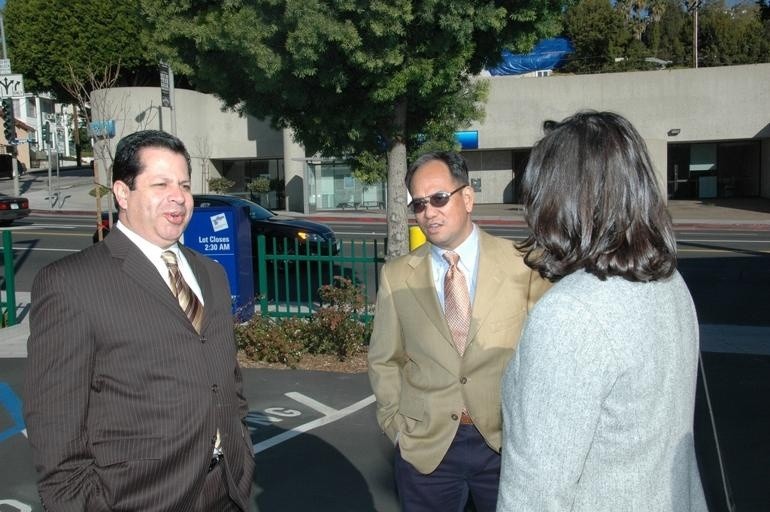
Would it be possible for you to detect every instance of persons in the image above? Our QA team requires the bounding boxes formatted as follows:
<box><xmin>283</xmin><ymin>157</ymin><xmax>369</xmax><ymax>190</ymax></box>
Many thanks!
<box><xmin>367</xmin><ymin>149</ymin><xmax>557</xmax><ymax>512</ymax></box>
<box><xmin>24</xmin><ymin>129</ymin><xmax>266</xmax><ymax>512</ymax></box>
<box><xmin>492</xmin><ymin>110</ymin><xmax>710</xmax><ymax>512</ymax></box>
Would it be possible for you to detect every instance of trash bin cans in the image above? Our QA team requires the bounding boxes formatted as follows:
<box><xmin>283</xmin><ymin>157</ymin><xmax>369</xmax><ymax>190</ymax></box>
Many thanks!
<box><xmin>182</xmin><ymin>204</ymin><xmax>255</xmax><ymax>325</ymax></box>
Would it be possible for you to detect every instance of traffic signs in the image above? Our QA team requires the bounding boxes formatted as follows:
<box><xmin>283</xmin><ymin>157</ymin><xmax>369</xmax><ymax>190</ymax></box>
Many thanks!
<box><xmin>0</xmin><ymin>58</ymin><xmax>12</xmax><ymax>75</ymax></box>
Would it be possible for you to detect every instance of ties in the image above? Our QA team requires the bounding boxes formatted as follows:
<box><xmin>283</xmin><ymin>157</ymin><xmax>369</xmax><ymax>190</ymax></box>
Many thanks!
<box><xmin>159</xmin><ymin>250</ymin><xmax>203</xmax><ymax>334</ymax></box>
<box><xmin>441</xmin><ymin>252</ymin><xmax>472</xmax><ymax>356</ymax></box>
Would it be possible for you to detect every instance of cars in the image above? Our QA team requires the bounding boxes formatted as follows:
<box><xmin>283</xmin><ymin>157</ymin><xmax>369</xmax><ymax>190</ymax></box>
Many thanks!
<box><xmin>0</xmin><ymin>190</ymin><xmax>33</xmax><ymax>228</ymax></box>
<box><xmin>0</xmin><ymin>153</ymin><xmax>25</xmax><ymax>180</ymax></box>
<box><xmin>90</xmin><ymin>191</ymin><xmax>342</xmax><ymax>281</ymax></box>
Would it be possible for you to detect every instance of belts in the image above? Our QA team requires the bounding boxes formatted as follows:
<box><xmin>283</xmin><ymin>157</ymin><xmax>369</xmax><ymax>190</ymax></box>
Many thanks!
<box><xmin>208</xmin><ymin>454</ymin><xmax>225</xmax><ymax>471</ymax></box>
<box><xmin>460</xmin><ymin>412</ymin><xmax>473</xmax><ymax>426</ymax></box>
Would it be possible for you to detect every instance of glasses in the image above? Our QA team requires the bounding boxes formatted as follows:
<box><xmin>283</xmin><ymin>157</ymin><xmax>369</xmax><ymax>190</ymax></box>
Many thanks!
<box><xmin>407</xmin><ymin>183</ymin><xmax>468</xmax><ymax>214</ymax></box>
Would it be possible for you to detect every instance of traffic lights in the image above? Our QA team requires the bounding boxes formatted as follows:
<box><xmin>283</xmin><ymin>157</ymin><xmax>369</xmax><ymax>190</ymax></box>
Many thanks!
<box><xmin>41</xmin><ymin>121</ymin><xmax>50</xmax><ymax>143</ymax></box>
<box><xmin>2</xmin><ymin>98</ymin><xmax>17</xmax><ymax>143</ymax></box>
